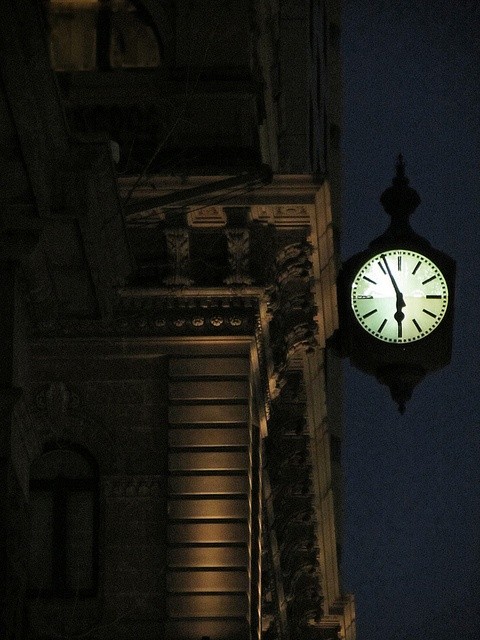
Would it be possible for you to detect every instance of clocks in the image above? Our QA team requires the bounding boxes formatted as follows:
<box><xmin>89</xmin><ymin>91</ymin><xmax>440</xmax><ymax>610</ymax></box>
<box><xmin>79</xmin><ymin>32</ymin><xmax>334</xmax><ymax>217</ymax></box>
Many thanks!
<box><xmin>328</xmin><ymin>154</ymin><xmax>457</xmax><ymax>413</ymax></box>
